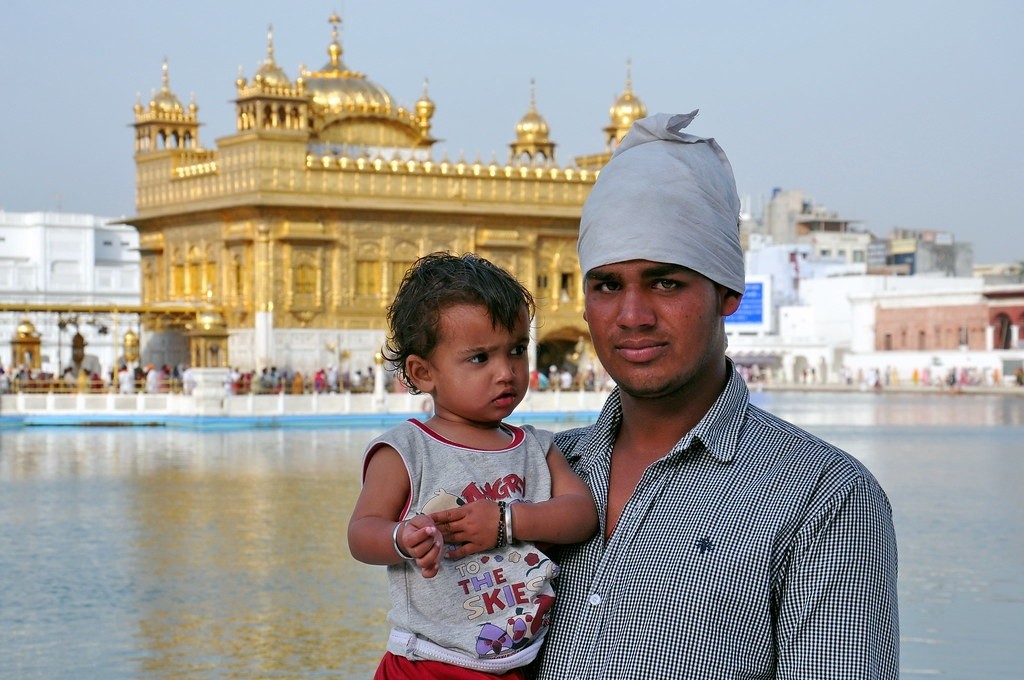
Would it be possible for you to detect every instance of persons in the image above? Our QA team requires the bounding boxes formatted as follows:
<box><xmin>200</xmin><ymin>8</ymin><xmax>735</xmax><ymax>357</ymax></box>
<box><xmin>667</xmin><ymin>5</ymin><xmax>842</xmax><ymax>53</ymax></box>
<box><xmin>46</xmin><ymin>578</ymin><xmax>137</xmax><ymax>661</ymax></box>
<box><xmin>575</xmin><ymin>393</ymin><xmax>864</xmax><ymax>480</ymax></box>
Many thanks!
<box><xmin>1</xmin><ymin>349</ymin><xmax>1024</xmax><ymax>393</ymax></box>
<box><xmin>342</xmin><ymin>250</ymin><xmax>601</xmax><ymax>680</ymax></box>
<box><xmin>520</xmin><ymin>107</ymin><xmax>900</xmax><ymax>680</ymax></box>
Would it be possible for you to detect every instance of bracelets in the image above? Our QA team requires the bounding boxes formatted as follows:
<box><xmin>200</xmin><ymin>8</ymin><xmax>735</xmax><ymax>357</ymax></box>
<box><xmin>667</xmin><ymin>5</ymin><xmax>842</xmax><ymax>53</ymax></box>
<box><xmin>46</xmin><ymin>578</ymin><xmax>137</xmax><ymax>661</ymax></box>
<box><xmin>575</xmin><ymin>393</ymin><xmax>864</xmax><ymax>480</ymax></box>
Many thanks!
<box><xmin>391</xmin><ymin>522</ymin><xmax>414</xmax><ymax>562</ymax></box>
<box><xmin>496</xmin><ymin>497</ymin><xmax>507</xmax><ymax>549</ymax></box>
<box><xmin>503</xmin><ymin>497</ymin><xmax>523</xmax><ymax>544</ymax></box>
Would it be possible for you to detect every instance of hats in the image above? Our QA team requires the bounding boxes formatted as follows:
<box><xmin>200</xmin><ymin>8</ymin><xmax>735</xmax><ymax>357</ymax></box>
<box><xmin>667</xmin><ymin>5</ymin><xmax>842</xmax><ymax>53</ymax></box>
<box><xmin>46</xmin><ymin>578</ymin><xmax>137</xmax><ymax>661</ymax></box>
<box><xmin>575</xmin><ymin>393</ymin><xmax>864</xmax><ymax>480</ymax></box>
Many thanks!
<box><xmin>576</xmin><ymin>108</ymin><xmax>745</xmax><ymax>295</ymax></box>
